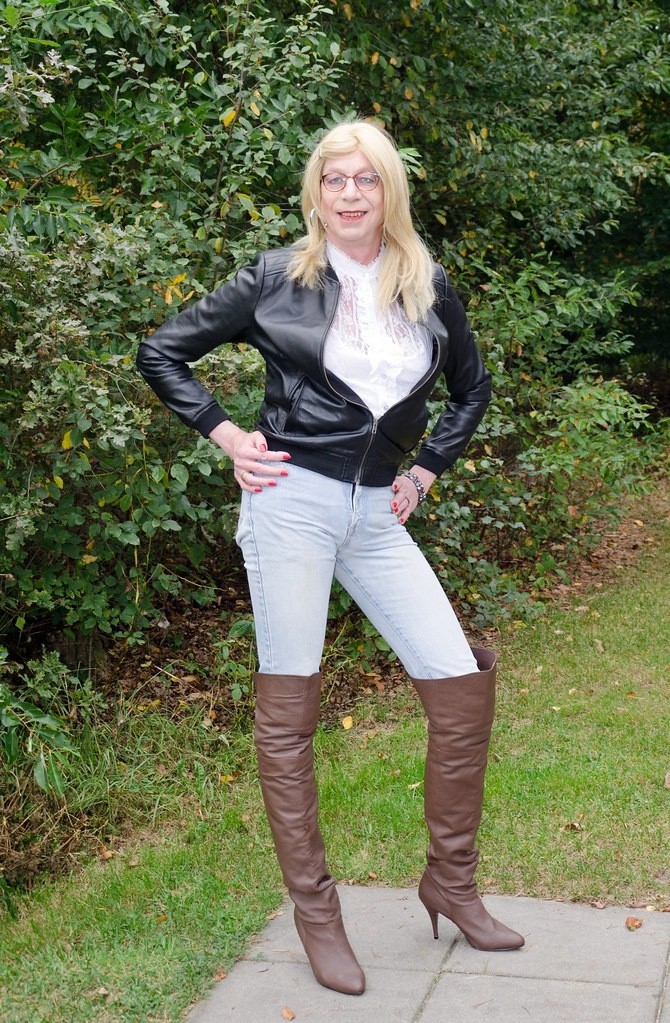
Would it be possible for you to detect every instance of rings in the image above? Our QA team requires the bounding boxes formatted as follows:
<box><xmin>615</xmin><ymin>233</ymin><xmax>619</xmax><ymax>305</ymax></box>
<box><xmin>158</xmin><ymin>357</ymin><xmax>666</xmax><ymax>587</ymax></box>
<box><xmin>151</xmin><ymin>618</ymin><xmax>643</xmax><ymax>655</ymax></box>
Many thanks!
<box><xmin>240</xmin><ymin>472</ymin><xmax>249</xmax><ymax>481</ymax></box>
<box><xmin>406</xmin><ymin>497</ymin><xmax>410</xmax><ymax>503</ymax></box>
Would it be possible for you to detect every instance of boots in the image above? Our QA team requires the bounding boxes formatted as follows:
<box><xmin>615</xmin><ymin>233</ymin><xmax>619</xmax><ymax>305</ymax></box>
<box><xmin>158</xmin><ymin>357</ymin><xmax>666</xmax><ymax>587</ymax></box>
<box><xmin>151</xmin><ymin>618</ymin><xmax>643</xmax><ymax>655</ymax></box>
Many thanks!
<box><xmin>404</xmin><ymin>646</ymin><xmax>526</xmax><ymax>952</ymax></box>
<box><xmin>251</xmin><ymin>668</ymin><xmax>368</xmax><ymax>997</ymax></box>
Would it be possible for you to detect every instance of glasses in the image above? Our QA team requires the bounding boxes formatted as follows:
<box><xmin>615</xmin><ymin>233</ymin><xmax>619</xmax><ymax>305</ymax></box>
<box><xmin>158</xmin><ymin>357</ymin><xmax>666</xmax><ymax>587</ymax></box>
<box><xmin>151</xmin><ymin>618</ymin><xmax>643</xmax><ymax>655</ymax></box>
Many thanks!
<box><xmin>320</xmin><ymin>172</ymin><xmax>380</xmax><ymax>193</ymax></box>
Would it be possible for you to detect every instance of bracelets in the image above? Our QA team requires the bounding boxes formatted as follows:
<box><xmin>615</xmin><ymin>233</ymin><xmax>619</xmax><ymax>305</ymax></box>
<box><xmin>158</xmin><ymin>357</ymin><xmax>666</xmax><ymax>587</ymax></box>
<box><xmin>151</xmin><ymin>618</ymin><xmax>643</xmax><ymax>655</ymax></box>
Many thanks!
<box><xmin>406</xmin><ymin>472</ymin><xmax>427</xmax><ymax>504</ymax></box>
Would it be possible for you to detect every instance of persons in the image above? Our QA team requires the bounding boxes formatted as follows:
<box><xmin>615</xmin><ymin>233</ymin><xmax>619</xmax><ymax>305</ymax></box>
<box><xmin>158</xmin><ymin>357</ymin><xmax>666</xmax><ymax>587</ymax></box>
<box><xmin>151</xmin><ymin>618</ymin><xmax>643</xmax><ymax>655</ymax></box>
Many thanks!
<box><xmin>134</xmin><ymin>123</ymin><xmax>525</xmax><ymax>998</ymax></box>
